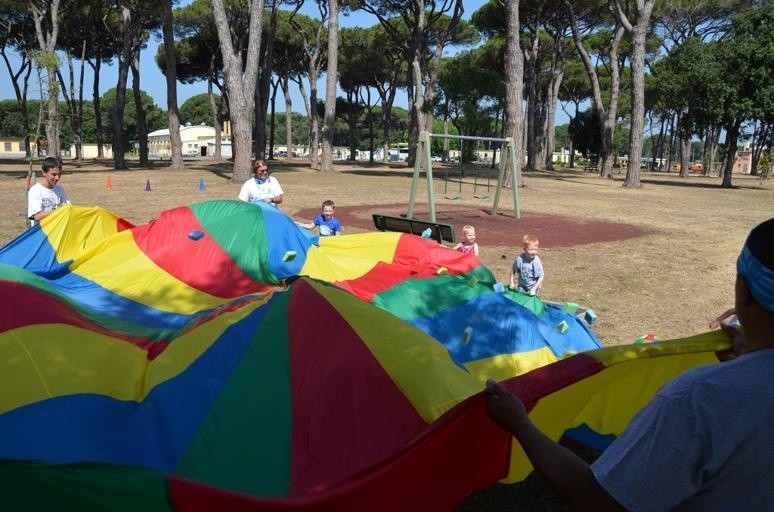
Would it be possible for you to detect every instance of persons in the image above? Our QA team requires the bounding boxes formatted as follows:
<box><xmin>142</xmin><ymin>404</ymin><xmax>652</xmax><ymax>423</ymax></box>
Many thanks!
<box><xmin>507</xmin><ymin>234</ymin><xmax>544</xmax><ymax>299</ymax></box>
<box><xmin>236</xmin><ymin>159</ymin><xmax>282</xmax><ymax>210</ymax></box>
<box><xmin>477</xmin><ymin>216</ymin><xmax>774</xmax><ymax>510</ymax></box>
<box><xmin>293</xmin><ymin>199</ymin><xmax>341</xmax><ymax>236</ymax></box>
<box><xmin>439</xmin><ymin>224</ymin><xmax>479</xmax><ymax>255</ymax></box>
<box><xmin>25</xmin><ymin>157</ymin><xmax>71</xmax><ymax>229</ymax></box>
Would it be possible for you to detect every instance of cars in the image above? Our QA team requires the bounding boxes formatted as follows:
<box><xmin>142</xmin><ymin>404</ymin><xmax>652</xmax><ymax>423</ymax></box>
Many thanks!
<box><xmin>181</xmin><ymin>150</ymin><xmax>198</xmax><ymax>157</ymax></box>
<box><xmin>263</xmin><ymin>149</ymin><xmax>304</xmax><ymax>159</ymax></box>
<box><xmin>430</xmin><ymin>156</ymin><xmax>459</xmax><ymax>163</ymax></box>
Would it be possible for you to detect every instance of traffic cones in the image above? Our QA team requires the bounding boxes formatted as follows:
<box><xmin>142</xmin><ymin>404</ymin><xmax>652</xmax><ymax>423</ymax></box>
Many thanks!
<box><xmin>144</xmin><ymin>179</ymin><xmax>152</xmax><ymax>193</ymax></box>
<box><xmin>104</xmin><ymin>175</ymin><xmax>114</xmax><ymax>192</ymax></box>
<box><xmin>198</xmin><ymin>177</ymin><xmax>206</xmax><ymax>193</ymax></box>
<box><xmin>22</xmin><ymin>175</ymin><xmax>32</xmax><ymax>189</ymax></box>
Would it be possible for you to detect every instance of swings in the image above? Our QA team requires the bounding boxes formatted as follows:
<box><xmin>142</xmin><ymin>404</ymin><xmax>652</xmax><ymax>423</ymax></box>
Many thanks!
<box><xmin>472</xmin><ymin>140</ymin><xmax>491</xmax><ymax>199</ymax></box>
<box><xmin>443</xmin><ymin>138</ymin><xmax>464</xmax><ymax>201</ymax></box>
